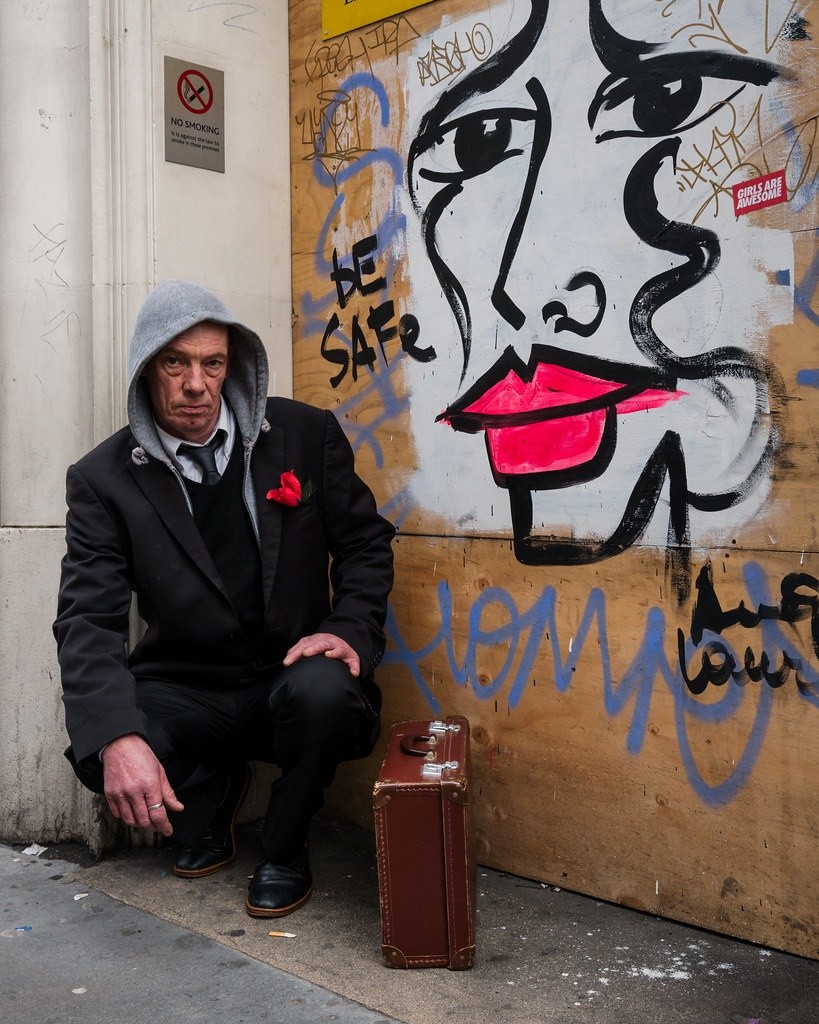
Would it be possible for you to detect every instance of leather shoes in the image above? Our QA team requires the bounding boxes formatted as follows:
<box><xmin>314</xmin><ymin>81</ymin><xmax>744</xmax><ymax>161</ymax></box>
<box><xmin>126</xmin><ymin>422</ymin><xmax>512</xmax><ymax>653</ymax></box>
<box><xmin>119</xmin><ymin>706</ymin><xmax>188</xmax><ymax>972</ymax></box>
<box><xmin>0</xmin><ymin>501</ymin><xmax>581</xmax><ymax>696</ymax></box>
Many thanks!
<box><xmin>246</xmin><ymin>831</ymin><xmax>314</xmax><ymax>917</ymax></box>
<box><xmin>173</xmin><ymin>761</ymin><xmax>252</xmax><ymax>877</ymax></box>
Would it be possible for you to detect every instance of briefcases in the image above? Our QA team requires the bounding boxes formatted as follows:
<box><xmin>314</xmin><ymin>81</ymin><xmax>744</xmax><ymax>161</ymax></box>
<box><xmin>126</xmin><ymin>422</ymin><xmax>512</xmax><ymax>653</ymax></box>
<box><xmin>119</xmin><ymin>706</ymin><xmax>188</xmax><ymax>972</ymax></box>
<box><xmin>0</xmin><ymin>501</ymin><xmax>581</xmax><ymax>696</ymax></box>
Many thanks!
<box><xmin>372</xmin><ymin>714</ymin><xmax>477</xmax><ymax>974</ymax></box>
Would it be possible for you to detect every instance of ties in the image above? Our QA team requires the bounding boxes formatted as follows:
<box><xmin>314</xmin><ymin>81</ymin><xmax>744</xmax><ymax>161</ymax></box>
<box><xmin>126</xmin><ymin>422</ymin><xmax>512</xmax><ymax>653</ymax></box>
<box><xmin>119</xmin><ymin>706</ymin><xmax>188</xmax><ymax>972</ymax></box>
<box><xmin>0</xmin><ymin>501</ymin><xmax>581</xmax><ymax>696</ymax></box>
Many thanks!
<box><xmin>176</xmin><ymin>429</ymin><xmax>229</xmax><ymax>487</ymax></box>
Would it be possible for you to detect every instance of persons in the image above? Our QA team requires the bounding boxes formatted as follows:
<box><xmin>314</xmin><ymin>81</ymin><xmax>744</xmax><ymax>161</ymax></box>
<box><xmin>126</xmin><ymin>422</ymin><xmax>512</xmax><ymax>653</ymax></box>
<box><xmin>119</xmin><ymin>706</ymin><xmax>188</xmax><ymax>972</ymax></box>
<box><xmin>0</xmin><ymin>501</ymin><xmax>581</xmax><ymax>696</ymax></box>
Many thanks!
<box><xmin>50</xmin><ymin>280</ymin><xmax>394</xmax><ymax>918</ymax></box>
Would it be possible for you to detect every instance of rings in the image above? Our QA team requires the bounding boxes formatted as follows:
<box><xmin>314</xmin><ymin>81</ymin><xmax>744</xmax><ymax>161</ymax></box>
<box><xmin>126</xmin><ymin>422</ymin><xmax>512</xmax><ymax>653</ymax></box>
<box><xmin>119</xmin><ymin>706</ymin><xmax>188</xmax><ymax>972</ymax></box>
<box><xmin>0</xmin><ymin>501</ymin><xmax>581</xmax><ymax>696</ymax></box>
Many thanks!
<box><xmin>148</xmin><ymin>802</ymin><xmax>163</xmax><ymax>811</ymax></box>
